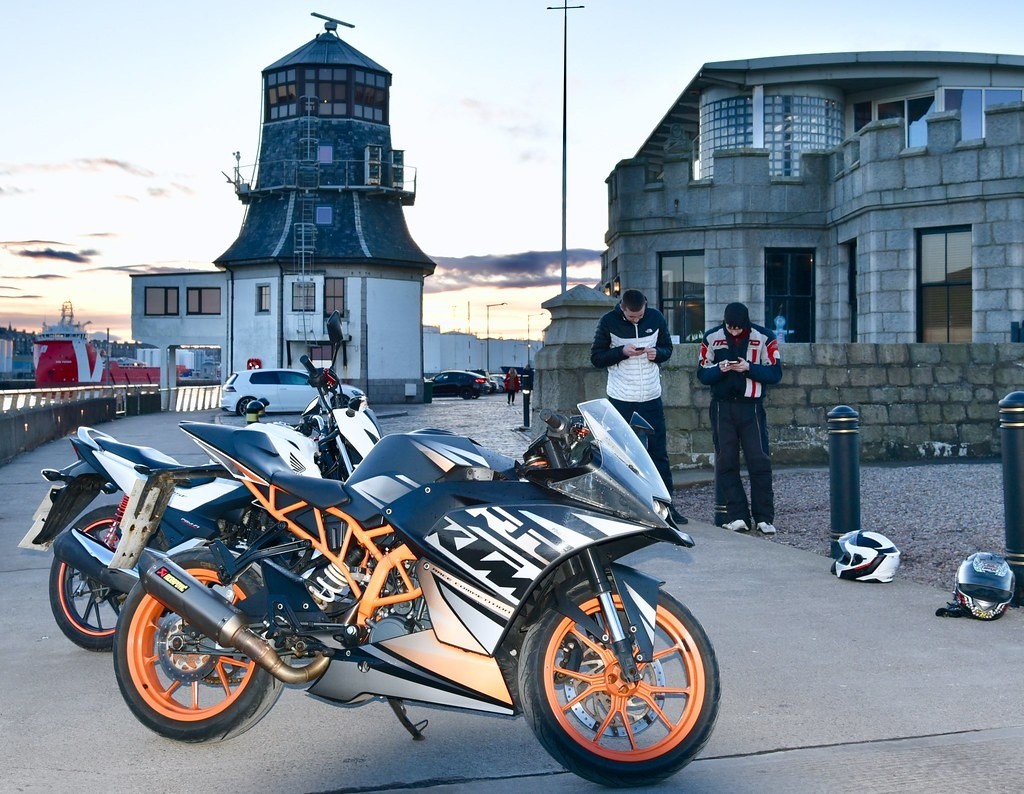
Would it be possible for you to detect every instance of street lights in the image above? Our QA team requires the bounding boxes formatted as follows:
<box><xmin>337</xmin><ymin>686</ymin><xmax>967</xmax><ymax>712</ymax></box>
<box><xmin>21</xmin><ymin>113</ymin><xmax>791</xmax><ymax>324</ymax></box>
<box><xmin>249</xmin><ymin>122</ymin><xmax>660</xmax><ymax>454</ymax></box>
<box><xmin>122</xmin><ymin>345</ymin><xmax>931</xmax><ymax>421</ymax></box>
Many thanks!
<box><xmin>486</xmin><ymin>301</ymin><xmax>508</xmax><ymax>374</ymax></box>
<box><xmin>527</xmin><ymin>312</ymin><xmax>544</xmax><ymax>367</ymax></box>
<box><xmin>546</xmin><ymin>0</ymin><xmax>586</xmax><ymax>294</ymax></box>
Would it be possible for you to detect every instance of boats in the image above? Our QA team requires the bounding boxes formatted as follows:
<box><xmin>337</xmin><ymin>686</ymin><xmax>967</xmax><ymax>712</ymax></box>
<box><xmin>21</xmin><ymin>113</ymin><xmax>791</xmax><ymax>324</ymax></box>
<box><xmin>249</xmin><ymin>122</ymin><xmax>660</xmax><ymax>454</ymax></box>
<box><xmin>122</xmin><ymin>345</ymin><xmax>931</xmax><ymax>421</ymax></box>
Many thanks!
<box><xmin>27</xmin><ymin>301</ymin><xmax>181</xmax><ymax>402</ymax></box>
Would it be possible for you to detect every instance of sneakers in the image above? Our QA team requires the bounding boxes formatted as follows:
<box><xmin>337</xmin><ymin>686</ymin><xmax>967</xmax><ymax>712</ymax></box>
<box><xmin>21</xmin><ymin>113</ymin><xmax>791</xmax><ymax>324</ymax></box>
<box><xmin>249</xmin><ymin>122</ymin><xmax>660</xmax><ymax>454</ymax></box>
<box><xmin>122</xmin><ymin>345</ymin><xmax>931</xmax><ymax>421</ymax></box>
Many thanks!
<box><xmin>756</xmin><ymin>522</ymin><xmax>776</xmax><ymax>534</ymax></box>
<box><xmin>723</xmin><ymin>519</ymin><xmax>749</xmax><ymax>531</ymax></box>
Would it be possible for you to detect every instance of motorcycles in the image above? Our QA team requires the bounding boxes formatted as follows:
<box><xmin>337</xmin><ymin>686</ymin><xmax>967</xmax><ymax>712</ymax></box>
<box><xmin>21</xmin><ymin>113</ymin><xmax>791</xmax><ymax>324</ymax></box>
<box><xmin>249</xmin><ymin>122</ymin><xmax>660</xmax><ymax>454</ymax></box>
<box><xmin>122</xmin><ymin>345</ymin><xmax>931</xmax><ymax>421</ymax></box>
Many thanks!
<box><xmin>110</xmin><ymin>399</ymin><xmax>722</xmax><ymax>788</ymax></box>
<box><xmin>16</xmin><ymin>309</ymin><xmax>385</xmax><ymax>653</ymax></box>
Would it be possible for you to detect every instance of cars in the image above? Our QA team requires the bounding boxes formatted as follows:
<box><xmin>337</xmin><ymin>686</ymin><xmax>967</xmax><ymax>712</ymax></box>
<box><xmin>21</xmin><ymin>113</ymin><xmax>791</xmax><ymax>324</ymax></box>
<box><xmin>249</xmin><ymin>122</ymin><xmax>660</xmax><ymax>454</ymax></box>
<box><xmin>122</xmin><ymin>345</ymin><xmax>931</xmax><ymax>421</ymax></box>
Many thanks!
<box><xmin>220</xmin><ymin>369</ymin><xmax>367</xmax><ymax>417</ymax></box>
<box><xmin>468</xmin><ymin>368</ymin><xmax>522</xmax><ymax>395</ymax></box>
<box><xmin>425</xmin><ymin>369</ymin><xmax>492</xmax><ymax>399</ymax></box>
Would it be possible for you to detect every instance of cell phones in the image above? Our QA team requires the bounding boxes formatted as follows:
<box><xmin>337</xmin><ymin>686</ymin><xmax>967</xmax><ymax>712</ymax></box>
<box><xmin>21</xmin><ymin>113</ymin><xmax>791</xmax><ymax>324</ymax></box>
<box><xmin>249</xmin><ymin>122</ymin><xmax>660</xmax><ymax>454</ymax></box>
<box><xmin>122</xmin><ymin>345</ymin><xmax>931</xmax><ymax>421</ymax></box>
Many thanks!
<box><xmin>635</xmin><ymin>346</ymin><xmax>646</xmax><ymax>351</ymax></box>
<box><xmin>729</xmin><ymin>361</ymin><xmax>740</xmax><ymax>365</ymax></box>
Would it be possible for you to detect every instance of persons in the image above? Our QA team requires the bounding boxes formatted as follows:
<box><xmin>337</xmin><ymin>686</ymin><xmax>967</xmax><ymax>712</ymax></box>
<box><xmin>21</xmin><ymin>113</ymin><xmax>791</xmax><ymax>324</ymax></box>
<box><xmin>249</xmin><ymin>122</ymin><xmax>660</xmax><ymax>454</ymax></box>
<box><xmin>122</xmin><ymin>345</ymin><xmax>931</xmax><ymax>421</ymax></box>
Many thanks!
<box><xmin>591</xmin><ymin>290</ymin><xmax>689</xmax><ymax>525</ymax></box>
<box><xmin>697</xmin><ymin>303</ymin><xmax>783</xmax><ymax>534</ymax></box>
<box><xmin>504</xmin><ymin>364</ymin><xmax>534</xmax><ymax>405</ymax></box>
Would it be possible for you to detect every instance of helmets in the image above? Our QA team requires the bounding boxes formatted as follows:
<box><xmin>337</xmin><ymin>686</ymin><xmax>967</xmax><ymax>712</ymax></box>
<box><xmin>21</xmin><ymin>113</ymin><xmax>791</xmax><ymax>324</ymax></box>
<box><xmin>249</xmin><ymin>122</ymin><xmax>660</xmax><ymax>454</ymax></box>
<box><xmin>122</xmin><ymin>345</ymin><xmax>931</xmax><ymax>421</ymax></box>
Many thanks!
<box><xmin>830</xmin><ymin>529</ymin><xmax>900</xmax><ymax>583</ymax></box>
<box><xmin>936</xmin><ymin>551</ymin><xmax>1015</xmax><ymax>620</ymax></box>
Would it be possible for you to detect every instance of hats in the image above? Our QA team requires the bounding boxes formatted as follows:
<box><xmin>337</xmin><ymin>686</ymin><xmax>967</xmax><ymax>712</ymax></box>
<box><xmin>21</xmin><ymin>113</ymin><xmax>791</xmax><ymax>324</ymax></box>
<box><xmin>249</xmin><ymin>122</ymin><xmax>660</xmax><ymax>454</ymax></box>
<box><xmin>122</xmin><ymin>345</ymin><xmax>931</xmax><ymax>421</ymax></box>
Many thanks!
<box><xmin>725</xmin><ymin>302</ymin><xmax>749</xmax><ymax>324</ymax></box>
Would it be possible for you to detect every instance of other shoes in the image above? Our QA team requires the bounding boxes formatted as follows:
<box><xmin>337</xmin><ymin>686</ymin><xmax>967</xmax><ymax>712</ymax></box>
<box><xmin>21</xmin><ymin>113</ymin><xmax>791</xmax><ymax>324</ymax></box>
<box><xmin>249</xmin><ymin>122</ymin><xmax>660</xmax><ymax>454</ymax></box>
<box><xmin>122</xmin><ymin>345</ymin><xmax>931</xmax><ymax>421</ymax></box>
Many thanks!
<box><xmin>662</xmin><ymin>502</ymin><xmax>688</xmax><ymax>524</ymax></box>
